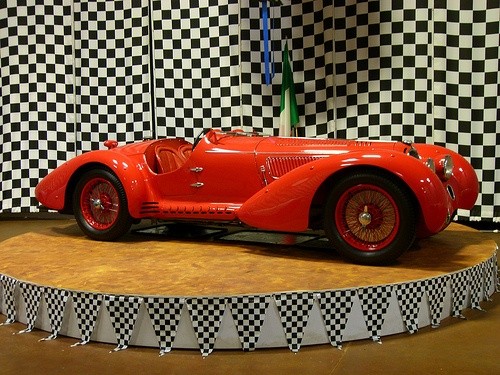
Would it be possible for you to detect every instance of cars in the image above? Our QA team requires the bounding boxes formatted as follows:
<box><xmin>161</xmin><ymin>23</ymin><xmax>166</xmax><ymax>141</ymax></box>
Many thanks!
<box><xmin>34</xmin><ymin>127</ymin><xmax>480</xmax><ymax>267</ymax></box>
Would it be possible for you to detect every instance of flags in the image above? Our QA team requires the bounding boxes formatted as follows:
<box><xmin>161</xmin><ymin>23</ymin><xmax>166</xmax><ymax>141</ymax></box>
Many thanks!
<box><xmin>278</xmin><ymin>40</ymin><xmax>301</xmax><ymax>137</ymax></box>
